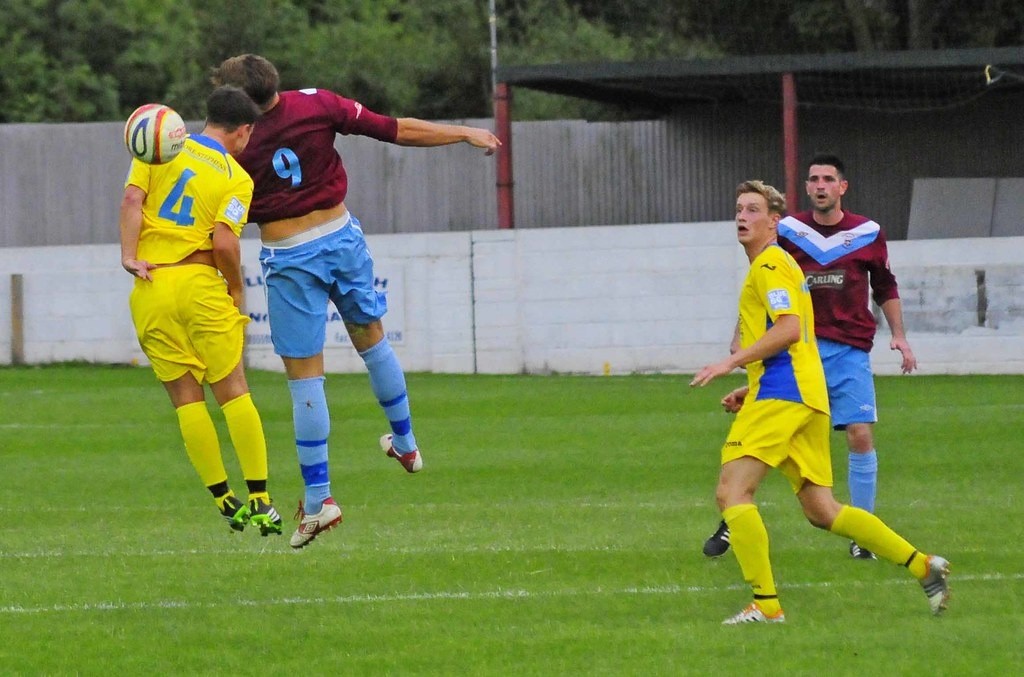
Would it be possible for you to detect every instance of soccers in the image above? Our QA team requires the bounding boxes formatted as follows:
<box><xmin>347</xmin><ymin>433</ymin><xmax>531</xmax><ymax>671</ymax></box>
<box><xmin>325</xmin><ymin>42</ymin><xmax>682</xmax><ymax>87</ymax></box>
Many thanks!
<box><xmin>123</xmin><ymin>103</ymin><xmax>187</xmax><ymax>165</ymax></box>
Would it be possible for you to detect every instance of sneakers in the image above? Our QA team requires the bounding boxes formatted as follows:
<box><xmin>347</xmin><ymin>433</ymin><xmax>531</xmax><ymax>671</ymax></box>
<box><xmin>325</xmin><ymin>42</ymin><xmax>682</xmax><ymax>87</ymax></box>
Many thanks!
<box><xmin>218</xmin><ymin>495</ymin><xmax>252</xmax><ymax>532</ymax></box>
<box><xmin>721</xmin><ymin>600</ymin><xmax>785</xmax><ymax>625</ymax></box>
<box><xmin>849</xmin><ymin>540</ymin><xmax>877</xmax><ymax>561</ymax></box>
<box><xmin>703</xmin><ymin>519</ymin><xmax>730</xmax><ymax>557</ymax></box>
<box><xmin>380</xmin><ymin>434</ymin><xmax>423</xmax><ymax>473</ymax></box>
<box><xmin>290</xmin><ymin>497</ymin><xmax>342</xmax><ymax>549</ymax></box>
<box><xmin>921</xmin><ymin>555</ymin><xmax>953</xmax><ymax>616</ymax></box>
<box><xmin>250</xmin><ymin>497</ymin><xmax>283</xmax><ymax>537</ymax></box>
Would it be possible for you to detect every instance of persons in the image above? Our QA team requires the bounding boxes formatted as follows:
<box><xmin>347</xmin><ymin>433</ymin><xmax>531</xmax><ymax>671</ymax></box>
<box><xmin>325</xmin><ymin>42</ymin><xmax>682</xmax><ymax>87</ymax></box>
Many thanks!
<box><xmin>703</xmin><ymin>151</ymin><xmax>917</xmax><ymax>561</ymax></box>
<box><xmin>688</xmin><ymin>180</ymin><xmax>953</xmax><ymax>625</ymax></box>
<box><xmin>120</xmin><ymin>84</ymin><xmax>281</xmax><ymax>539</ymax></box>
<box><xmin>207</xmin><ymin>53</ymin><xmax>502</xmax><ymax>550</ymax></box>
